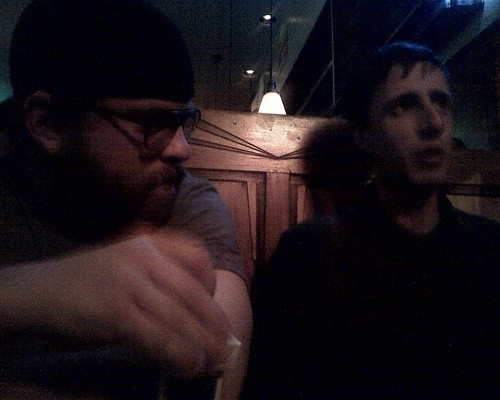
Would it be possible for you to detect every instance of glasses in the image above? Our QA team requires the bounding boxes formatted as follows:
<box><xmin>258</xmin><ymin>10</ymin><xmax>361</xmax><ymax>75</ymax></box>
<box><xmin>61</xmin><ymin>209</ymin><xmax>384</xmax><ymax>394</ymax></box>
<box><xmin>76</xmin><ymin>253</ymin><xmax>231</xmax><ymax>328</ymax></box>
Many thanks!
<box><xmin>77</xmin><ymin>98</ymin><xmax>201</xmax><ymax>150</ymax></box>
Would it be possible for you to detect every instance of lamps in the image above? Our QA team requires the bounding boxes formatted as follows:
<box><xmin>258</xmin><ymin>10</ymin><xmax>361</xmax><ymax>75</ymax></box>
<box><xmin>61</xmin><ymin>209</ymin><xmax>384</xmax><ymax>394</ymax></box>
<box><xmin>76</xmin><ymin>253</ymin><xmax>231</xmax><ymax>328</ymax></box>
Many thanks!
<box><xmin>258</xmin><ymin>79</ymin><xmax>287</xmax><ymax>116</ymax></box>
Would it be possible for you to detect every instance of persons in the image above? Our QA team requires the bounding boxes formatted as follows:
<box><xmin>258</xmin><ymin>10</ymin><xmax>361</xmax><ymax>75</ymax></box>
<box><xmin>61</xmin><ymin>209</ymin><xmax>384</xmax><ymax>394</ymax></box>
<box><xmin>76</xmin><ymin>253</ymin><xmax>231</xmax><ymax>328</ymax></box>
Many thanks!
<box><xmin>0</xmin><ymin>0</ymin><xmax>252</xmax><ymax>400</ymax></box>
<box><xmin>242</xmin><ymin>43</ymin><xmax>500</xmax><ymax>400</ymax></box>
